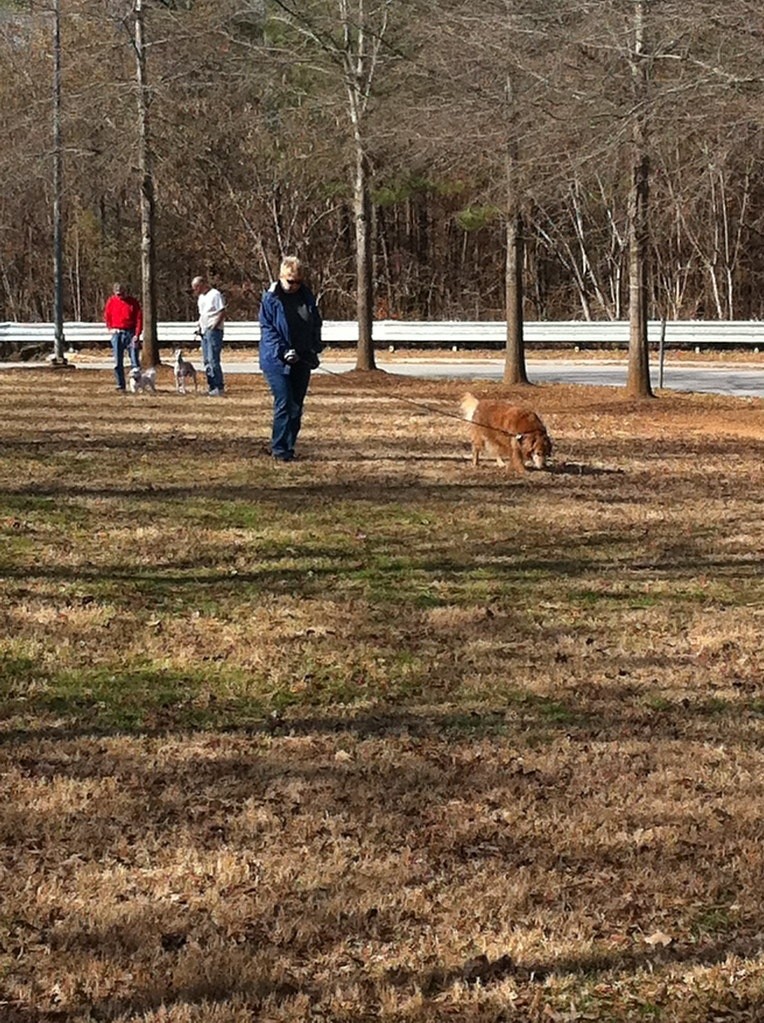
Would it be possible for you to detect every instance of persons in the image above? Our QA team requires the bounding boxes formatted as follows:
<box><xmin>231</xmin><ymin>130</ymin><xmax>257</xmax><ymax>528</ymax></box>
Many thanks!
<box><xmin>259</xmin><ymin>255</ymin><xmax>323</xmax><ymax>461</ymax></box>
<box><xmin>191</xmin><ymin>277</ymin><xmax>226</xmax><ymax>396</ymax></box>
<box><xmin>104</xmin><ymin>283</ymin><xmax>143</xmax><ymax>390</ymax></box>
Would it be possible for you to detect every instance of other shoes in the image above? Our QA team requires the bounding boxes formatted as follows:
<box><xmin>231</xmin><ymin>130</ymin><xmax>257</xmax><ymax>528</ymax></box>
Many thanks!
<box><xmin>115</xmin><ymin>385</ymin><xmax>124</xmax><ymax>390</ymax></box>
<box><xmin>208</xmin><ymin>386</ymin><xmax>225</xmax><ymax>395</ymax></box>
<box><xmin>272</xmin><ymin>453</ymin><xmax>305</xmax><ymax>463</ymax></box>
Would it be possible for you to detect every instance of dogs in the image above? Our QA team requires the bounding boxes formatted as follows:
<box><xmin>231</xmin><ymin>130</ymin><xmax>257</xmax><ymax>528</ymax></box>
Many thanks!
<box><xmin>173</xmin><ymin>349</ymin><xmax>197</xmax><ymax>394</ymax></box>
<box><xmin>127</xmin><ymin>367</ymin><xmax>157</xmax><ymax>395</ymax></box>
<box><xmin>460</xmin><ymin>391</ymin><xmax>552</xmax><ymax>473</ymax></box>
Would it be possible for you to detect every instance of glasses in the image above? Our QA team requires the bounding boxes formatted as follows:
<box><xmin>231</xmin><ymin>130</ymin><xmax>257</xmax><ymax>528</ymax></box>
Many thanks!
<box><xmin>286</xmin><ymin>279</ymin><xmax>302</xmax><ymax>285</ymax></box>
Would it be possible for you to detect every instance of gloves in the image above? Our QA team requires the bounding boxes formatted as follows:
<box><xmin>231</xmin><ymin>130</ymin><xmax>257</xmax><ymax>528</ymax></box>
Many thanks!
<box><xmin>283</xmin><ymin>348</ymin><xmax>300</xmax><ymax>363</ymax></box>
<box><xmin>308</xmin><ymin>351</ymin><xmax>321</xmax><ymax>369</ymax></box>
